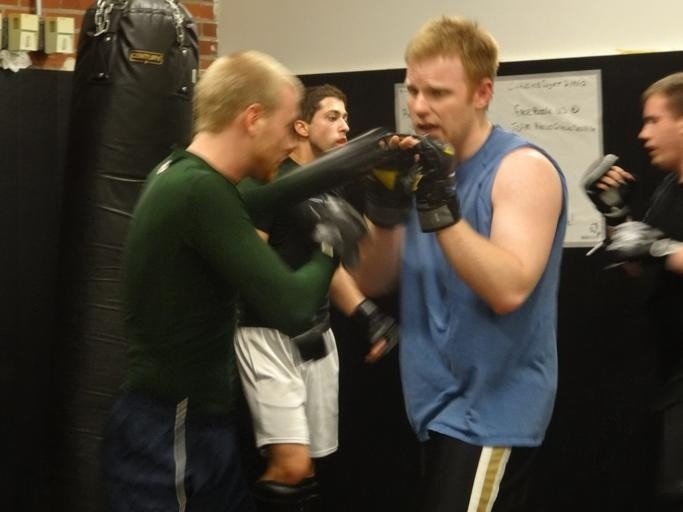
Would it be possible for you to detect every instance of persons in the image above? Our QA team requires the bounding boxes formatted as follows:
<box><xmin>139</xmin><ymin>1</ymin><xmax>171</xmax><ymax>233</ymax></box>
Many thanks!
<box><xmin>583</xmin><ymin>68</ymin><xmax>683</xmax><ymax>512</ymax></box>
<box><xmin>100</xmin><ymin>50</ymin><xmax>394</xmax><ymax>512</ymax></box>
<box><xmin>230</xmin><ymin>81</ymin><xmax>412</xmax><ymax>512</ymax></box>
<box><xmin>379</xmin><ymin>20</ymin><xmax>569</xmax><ymax>512</ymax></box>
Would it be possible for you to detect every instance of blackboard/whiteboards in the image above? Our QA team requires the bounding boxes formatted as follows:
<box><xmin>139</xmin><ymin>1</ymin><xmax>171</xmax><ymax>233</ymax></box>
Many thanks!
<box><xmin>394</xmin><ymin>69</ymin><xmax>607</xmax><ymax>248</ymax></box>
